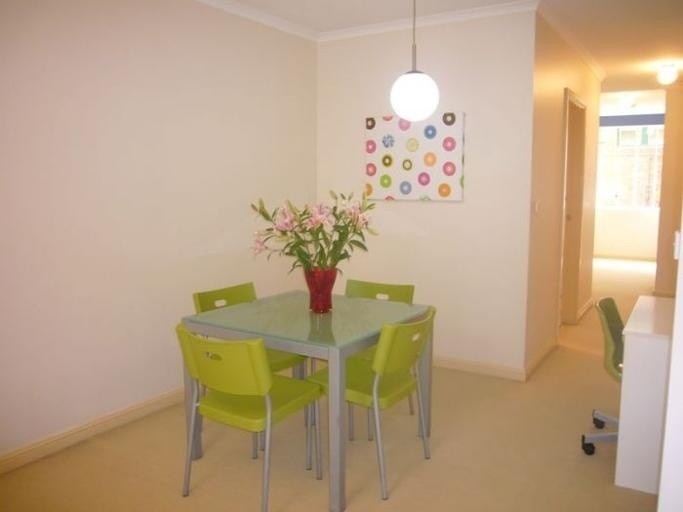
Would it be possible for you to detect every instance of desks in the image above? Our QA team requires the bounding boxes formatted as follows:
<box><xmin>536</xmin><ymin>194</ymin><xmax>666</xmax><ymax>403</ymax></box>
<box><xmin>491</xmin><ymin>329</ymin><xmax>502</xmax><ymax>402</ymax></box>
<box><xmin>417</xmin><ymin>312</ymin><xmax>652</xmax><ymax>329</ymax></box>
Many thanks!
<box><xmin>613</xmin><ymin>294</ymin><xmax>675</xmax><ymax>495</ymax></box>
<box><xmin>181</xmin><ymin>289</ymin><xmax>433</xmax><ymax>512</ymax></box>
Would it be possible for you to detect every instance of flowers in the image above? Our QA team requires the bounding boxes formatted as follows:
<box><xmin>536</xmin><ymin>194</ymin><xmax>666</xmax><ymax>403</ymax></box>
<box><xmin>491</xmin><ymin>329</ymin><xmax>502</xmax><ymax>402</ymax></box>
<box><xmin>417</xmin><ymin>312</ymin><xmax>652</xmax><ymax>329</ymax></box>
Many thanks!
<box><xmin>250</xmin><ymin>189</ymin><xmax>376</xmax><ymax>269</ymax></box>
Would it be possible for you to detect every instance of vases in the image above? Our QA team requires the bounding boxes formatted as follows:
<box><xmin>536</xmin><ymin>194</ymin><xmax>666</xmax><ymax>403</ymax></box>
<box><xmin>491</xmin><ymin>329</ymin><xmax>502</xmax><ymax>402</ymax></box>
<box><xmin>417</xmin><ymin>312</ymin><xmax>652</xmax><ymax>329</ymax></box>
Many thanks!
<box><xmin>303</xmin><ymin>268</ymin><xmax>336</xmax><ymax>313</ymax></box>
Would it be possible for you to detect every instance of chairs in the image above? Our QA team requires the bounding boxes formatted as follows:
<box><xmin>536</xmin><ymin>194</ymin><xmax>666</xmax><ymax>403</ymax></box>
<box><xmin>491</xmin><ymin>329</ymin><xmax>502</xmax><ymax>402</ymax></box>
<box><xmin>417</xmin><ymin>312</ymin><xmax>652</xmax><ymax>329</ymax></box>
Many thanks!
<box><xmin>582</xmin><ymin>299</ymin><xmax>624</xmax><ymax>454</ymax></box>
<box><xmin>306</xmin><ymin>309</ymin><xmax>435</xmax><ymax>500</ymax></box>
<box><xmin>193</xmin><ymin>282</ymin><xmax>317</xmax><ymax>450</ymax></box>
<box><xmin>176</xmin><ymin>325</ymin><xmax>322</xmax><ymax>512</ymax></box>
<box><xmin>309</xmin><ymin>280</ymin><xmax>415</xmax><ymax>441</ymax></box>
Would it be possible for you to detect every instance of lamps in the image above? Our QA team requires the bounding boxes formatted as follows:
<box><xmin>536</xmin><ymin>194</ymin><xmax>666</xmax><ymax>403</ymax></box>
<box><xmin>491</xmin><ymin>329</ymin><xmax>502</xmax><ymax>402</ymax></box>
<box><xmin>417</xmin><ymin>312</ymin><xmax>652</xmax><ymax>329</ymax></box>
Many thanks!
<box><xmin>389</xmin><ymin>1</ymin><xmax>439</xmax><ymax>123</ymax></box>
